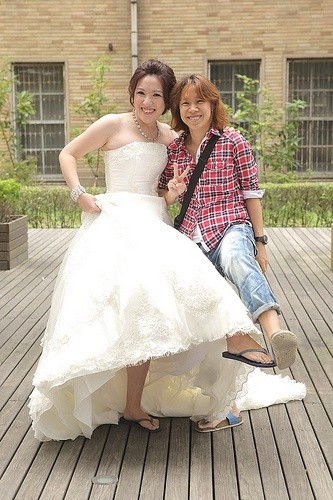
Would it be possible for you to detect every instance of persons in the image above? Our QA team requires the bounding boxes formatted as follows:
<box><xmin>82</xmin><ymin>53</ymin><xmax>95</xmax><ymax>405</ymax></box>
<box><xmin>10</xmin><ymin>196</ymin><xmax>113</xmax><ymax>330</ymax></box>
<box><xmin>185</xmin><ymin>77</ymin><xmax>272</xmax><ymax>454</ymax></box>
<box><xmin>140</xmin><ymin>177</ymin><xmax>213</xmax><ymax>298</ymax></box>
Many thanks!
<box><xmin>28</xmin><ymin>59</ymin><xmax>306</xmax><ymax>441</ymax></box>
<box><xmin>156</xmin><ymin>74</ymin><xmax>299</xmax><ymax>431</ymax></box>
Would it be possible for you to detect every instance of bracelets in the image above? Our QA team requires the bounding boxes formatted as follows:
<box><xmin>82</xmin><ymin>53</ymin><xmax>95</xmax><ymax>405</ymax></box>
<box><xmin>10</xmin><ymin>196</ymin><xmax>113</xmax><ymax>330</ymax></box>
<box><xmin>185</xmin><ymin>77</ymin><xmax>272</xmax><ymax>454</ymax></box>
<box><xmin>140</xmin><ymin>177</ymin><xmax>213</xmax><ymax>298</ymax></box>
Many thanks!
<box><xmin>70</xmin><ymin>184</ymin><xmax>85</xmax><ymax>203</ymax></box>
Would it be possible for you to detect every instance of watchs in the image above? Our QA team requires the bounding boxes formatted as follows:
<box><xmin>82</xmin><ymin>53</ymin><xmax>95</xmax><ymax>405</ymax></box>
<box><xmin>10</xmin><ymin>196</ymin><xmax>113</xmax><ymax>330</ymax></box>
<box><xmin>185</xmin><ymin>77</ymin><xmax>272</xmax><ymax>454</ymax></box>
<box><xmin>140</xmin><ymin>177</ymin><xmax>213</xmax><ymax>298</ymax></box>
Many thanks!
<box><xmin>254</xmin><ymin>235</ymin><xmax>269</xmax><ymax>245</ymax></box>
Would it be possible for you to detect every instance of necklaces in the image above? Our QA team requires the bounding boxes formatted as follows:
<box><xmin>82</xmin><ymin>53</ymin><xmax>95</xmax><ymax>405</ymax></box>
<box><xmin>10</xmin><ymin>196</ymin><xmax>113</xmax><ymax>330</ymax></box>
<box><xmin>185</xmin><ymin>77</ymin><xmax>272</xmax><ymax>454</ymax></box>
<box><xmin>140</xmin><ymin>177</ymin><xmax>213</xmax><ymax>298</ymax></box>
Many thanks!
<box><xmin>132</xmin><ymin>109</ymin><xmax>161</xmax><ymax>143</ymax></box>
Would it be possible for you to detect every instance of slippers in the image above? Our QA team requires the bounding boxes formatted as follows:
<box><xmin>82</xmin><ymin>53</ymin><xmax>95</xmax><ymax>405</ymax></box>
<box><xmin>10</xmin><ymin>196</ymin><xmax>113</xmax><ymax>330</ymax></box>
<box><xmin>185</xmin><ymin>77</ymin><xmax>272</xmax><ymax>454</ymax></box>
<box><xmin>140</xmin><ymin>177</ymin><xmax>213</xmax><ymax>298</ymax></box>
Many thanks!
<box><xmin>195</xmin><ymin>412</ymin><xmax>244</xmax><ymax>432</ymax></box>
<box><xmin>120</xmin><ymin>413</ymin><xmax>162</xmax><ymax>433</ymax></box>
<box><xmin>271</xmin><ymin>330</ymin><xmax>298</xmax><ymax>369</ymax></box>
<box><xmin>222</xmin><ymin>347</ymin><xmax>277</xmax><ymax>368</ymax></box>
